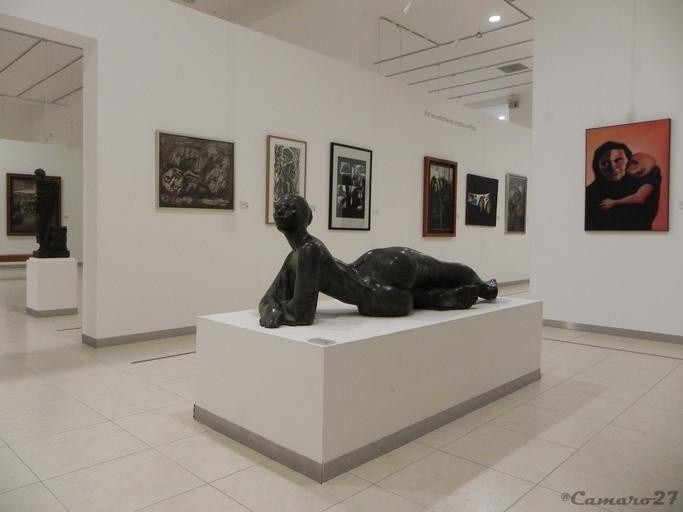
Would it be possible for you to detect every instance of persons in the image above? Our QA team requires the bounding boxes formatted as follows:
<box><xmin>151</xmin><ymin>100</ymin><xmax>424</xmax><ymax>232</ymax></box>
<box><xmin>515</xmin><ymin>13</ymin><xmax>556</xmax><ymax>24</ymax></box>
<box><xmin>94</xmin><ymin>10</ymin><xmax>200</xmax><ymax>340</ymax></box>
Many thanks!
<box><xmin>259</xmin><ymin>195</ymin><xmax>498</xmax><ymax>328</ymax></box>
<box><xmin>586</xmin><ymin>141</ymin><xmax>662</xmax><ymax>231</ymax></box>
<box><xmin>33</xmin><ymin>169</ymin><xmax>56</xmax><ymax>256</ymax></box>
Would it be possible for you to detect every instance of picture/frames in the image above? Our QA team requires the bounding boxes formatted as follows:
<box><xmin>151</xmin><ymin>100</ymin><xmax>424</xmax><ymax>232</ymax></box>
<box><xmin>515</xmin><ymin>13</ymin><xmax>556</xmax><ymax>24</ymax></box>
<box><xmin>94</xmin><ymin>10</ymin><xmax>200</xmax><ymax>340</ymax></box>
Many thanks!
<box><xmin>7</xmin><ymin>173</ymin><xmax>62</xmax><ymax>235</ymax></box>
<box><xmin>586</xmin><ymin>118</ymin><xmax>672</xmax><ymax>232</ymax></box>
<box><xmin>425</xmin><ymin>156</ymin><xmax>527</xmax><ymax>237</ymax></box>
<box><xmin>266</xmin><ymin>133</ymin><xmax>371</xmax><ymax>229</ymax></box>
<box><xmin>156</xmin><ymin>131</ymin><xmax>240</xmax><ymax>215</ymax></box>
